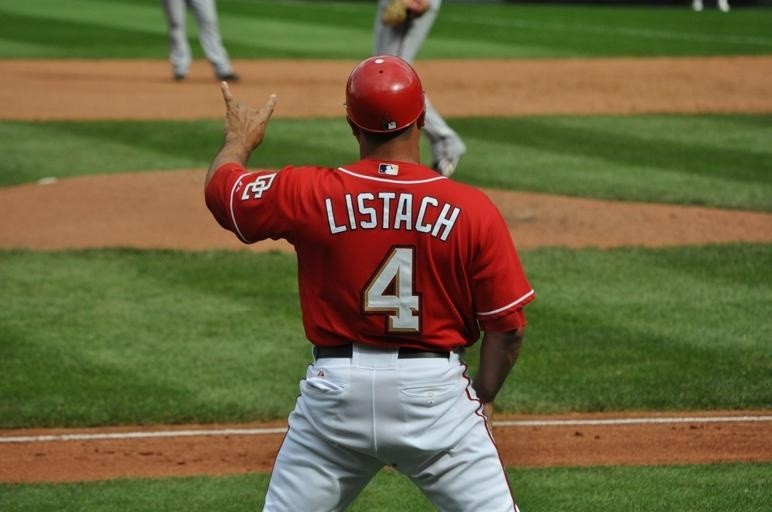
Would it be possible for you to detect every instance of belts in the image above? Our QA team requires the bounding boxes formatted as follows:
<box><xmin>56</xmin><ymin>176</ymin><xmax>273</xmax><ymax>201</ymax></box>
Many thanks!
<box><xmin>310</xmin><ymin>345</ymin><xmax>450</xmax><ymax>359</ymax></box>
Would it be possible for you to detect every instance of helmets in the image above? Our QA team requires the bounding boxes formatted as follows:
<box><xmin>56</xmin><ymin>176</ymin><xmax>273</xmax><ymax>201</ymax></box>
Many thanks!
<box><xmin>345</xmin><ymin>55</ymin><xmax>424</xmax><ymax>132</ymax></box>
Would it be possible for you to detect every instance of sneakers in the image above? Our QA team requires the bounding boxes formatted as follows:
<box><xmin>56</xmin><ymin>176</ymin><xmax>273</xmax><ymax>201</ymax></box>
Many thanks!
<box><xmin>431</xmin><ymin>154</ymin><xmax>460</xmax><ymax>179</ymax></box>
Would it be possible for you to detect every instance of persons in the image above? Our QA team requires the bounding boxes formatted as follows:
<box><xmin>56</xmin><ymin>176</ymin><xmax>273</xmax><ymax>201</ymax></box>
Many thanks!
<box><xmin>202</xmin><ymin>53</ymin><xmax>537</xmax><ymax>512</ymax></box>
<box><xmin>370</xmin><ymin>0</ymin><xmax>468</xmax><ymax>179</ymax></box>
<box><xmin>162</xmin><ymin>0</ymin><xmax>240</xmax><ymax>80</ymax></box>
<box><xmin>690</xmin><ymin>0</ymin><xmax>731</xmax><ymax>13</ymax></box>
